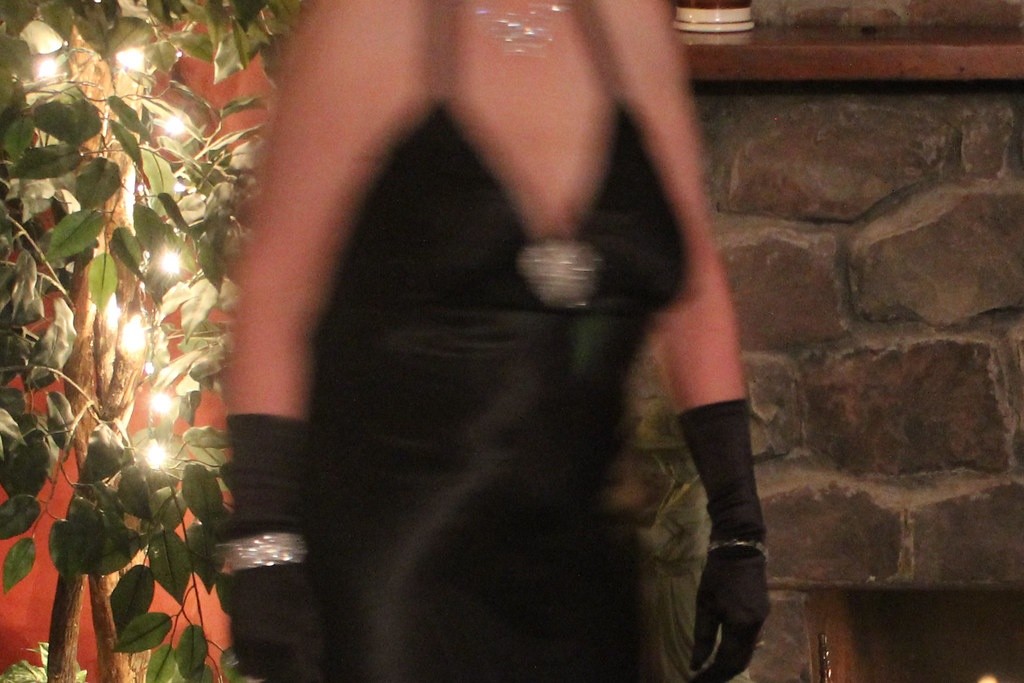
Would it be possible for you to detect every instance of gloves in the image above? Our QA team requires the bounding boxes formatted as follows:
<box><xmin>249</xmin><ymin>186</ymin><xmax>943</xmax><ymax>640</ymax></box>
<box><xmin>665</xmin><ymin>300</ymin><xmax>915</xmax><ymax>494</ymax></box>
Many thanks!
<box><xmin>223</xmin><ymin>413</ymin><xmax>324</xmax><ymax>683</ymax></box>
<box><xmin>683</xmin><ymin>396</ymin><xmax>768</xmax><ymax>683</ymax></box>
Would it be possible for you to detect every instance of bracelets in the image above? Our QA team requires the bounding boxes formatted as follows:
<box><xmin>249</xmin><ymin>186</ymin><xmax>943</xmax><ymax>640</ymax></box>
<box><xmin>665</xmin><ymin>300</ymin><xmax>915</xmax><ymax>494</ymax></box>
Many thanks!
<box><xmin>214</xmin><ymin>534</ymin><xmax>307</xmax><ymax>570</ymax></box>
<box><xmin>709</xmin><ymin>541</ymin><xmax>766</xmax><ymax>549</ymax></box>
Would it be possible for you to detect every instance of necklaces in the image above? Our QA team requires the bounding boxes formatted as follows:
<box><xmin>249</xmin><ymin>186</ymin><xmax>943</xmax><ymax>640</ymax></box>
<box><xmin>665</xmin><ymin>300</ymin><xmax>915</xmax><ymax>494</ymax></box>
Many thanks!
<box><xmin>473</xmin><ymin>7</ymin><xmax>567</xmax><ymax>54</ymax></box>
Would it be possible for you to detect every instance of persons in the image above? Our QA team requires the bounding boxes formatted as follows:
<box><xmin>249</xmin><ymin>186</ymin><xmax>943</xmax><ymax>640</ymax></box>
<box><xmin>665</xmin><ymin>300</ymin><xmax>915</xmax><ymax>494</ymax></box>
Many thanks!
<box><xmin>217</xmin><ymin>1</ymin><xmax>770</xmax><ymax>682</ymax></box>
